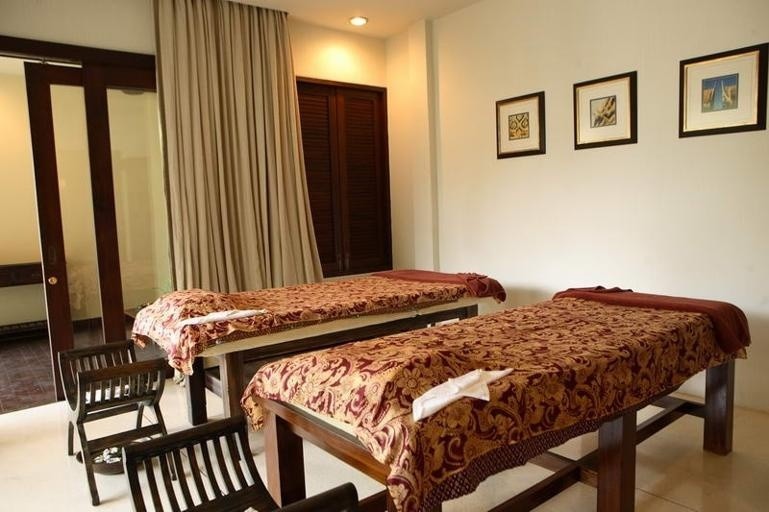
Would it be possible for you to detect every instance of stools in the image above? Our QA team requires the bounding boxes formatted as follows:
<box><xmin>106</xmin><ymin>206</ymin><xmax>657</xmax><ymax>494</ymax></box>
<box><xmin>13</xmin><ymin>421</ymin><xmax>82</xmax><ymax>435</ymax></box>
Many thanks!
<box><xmin>118</xmin><ymin>413</ymin><xmax>362</xmax><ymax>512</ymax></box>
<box><xmin>56</xmin><ymin>338</ymin><xmax>178</xmax><ymax>506</ymax></box>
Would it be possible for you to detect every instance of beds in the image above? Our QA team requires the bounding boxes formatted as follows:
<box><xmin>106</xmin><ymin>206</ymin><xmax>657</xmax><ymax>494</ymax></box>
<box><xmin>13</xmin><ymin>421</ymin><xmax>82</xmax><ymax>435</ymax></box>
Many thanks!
<box><xmin>250</xmin><ymin>286</ymin><xmax>742</xmax><ymax>512</ymax></box>
<box><xmin>136</xmin><ymin>267</ymin><xmax>502</xmax><ymax>458</ymax></box>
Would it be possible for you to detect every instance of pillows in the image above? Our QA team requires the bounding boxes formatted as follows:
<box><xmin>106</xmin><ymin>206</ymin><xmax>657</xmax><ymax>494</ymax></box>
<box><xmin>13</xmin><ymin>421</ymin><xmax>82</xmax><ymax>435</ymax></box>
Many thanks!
<box><xmin>149</xmin><ymin>287</ymin><xmax>236</xmax><ymax>327</ymax></box>
<box><xmin>272</xmin><ymin>340</ymin><xmax>490</xmax><ymax>435</ymax></box>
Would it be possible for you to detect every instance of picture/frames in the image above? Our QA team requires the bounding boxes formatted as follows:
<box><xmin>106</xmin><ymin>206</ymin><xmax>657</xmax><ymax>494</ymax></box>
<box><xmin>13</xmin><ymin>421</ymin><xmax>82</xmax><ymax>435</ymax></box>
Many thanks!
<box><xmin>572</xmin><ymin>69</ymin><xmax>638</xmax><ymax>150</ymax></box>
<box><xmin>495</xmin><ymin>89</ymin><xmax>546</xmax><ymax>160</ymax></box>
<box><xmin>678</xmin><ymin>41</ymin><xmax>768</xmax><ymax>138</ymax></box>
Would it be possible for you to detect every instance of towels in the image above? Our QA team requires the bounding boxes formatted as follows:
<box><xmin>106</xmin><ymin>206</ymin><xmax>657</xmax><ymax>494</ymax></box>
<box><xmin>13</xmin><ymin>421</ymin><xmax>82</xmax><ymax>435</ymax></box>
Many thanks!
<box><xmin>172</xmin><ymin>307</ymin><xmax>270</xmax><ymax>347</ymax></box>
<box><xmin>412</xmin><ymin>366</ymin><xmax>515</xmax><ymax>423</ymax></box>
